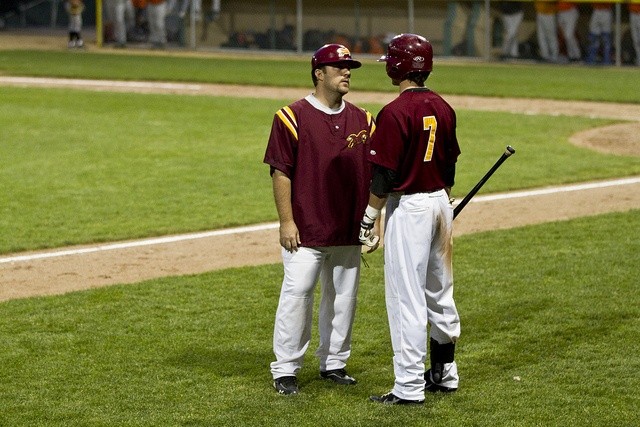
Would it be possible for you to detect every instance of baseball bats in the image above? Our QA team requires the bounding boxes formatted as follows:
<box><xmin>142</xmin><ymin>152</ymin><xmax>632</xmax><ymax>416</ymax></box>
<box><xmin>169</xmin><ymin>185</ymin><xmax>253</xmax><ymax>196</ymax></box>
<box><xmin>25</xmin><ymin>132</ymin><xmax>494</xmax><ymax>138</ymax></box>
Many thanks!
<box><xmin>454</xmin><ymin>143</ymin><xmax>515</xmax><ymax>220</ymax></box>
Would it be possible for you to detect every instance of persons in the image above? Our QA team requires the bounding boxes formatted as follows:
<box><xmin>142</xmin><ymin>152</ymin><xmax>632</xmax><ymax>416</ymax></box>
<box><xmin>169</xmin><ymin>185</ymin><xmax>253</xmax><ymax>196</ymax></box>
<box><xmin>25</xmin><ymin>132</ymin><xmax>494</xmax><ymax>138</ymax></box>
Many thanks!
<box><xmin>104</xmin><ymin>0</ymin><xmax>222</xmax><ymax>48</ymax></box>
<box><xmin>359</xmin><ymin>33</ymin><xmax>462</xmax><ymax>403</ymax></box>
<box><xmin>65</xmin><ymin>1</ymin><xmax>85</xmax><ymax>47</ymax></box>
<box><xmin>264</xmin><ymin>42</ymin><xmax>381</xmax><ymax>394</ymax></box>
<box><xmin>494</xmin><ymin>0</ymin><xmax>640</xmax><ymax>67</ymax></box>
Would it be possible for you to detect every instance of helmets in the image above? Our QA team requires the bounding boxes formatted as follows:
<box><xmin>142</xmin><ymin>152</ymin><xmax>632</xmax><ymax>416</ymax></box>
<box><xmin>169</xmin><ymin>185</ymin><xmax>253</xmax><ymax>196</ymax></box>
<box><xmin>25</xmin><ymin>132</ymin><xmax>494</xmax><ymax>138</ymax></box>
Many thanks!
<box><xmin>376</xmin><ymin>34</ymin><xmax>434</xmax><ymax>85</ymax></box>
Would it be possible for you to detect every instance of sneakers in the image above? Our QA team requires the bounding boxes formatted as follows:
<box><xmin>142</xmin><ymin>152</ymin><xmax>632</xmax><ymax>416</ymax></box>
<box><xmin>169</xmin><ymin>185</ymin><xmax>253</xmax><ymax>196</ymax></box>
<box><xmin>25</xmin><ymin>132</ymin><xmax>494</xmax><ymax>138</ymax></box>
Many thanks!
<box><xmin>321</xmin><ymin>367</ymin><xmax>356</xmax><ymax>385</ymax></box>
<box><xmin>422</xmin><ymin>369</ymin><xmax>461</xmax><ymax>392</ymax></box>
<box><xmin>370</xmin><ymin>394</ymin><xmax>426</xmax><ymax>406</ymax></box>
<box><xmin>274</xmin><ymin>375</ymin><xmax>301</xmax><ymax>394</ymax></box>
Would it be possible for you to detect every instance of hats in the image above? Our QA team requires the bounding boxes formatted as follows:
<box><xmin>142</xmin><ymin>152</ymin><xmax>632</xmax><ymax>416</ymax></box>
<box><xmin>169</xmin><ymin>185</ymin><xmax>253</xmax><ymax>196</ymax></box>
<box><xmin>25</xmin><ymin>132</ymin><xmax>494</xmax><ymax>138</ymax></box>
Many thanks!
<box><xmin>311</xmin><ymin>45</ymin><xmax>363</xmax><ymax>71</ymax></box>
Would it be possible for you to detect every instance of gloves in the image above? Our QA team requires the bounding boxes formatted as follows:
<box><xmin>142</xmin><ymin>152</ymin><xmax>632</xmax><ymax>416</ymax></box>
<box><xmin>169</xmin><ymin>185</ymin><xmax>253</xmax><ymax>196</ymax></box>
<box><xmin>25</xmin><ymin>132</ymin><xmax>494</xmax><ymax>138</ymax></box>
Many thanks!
<box><xmin>359</xmin><ymin>211</ymin><xmax>380</xmax><ymax>246</ymax></box>
